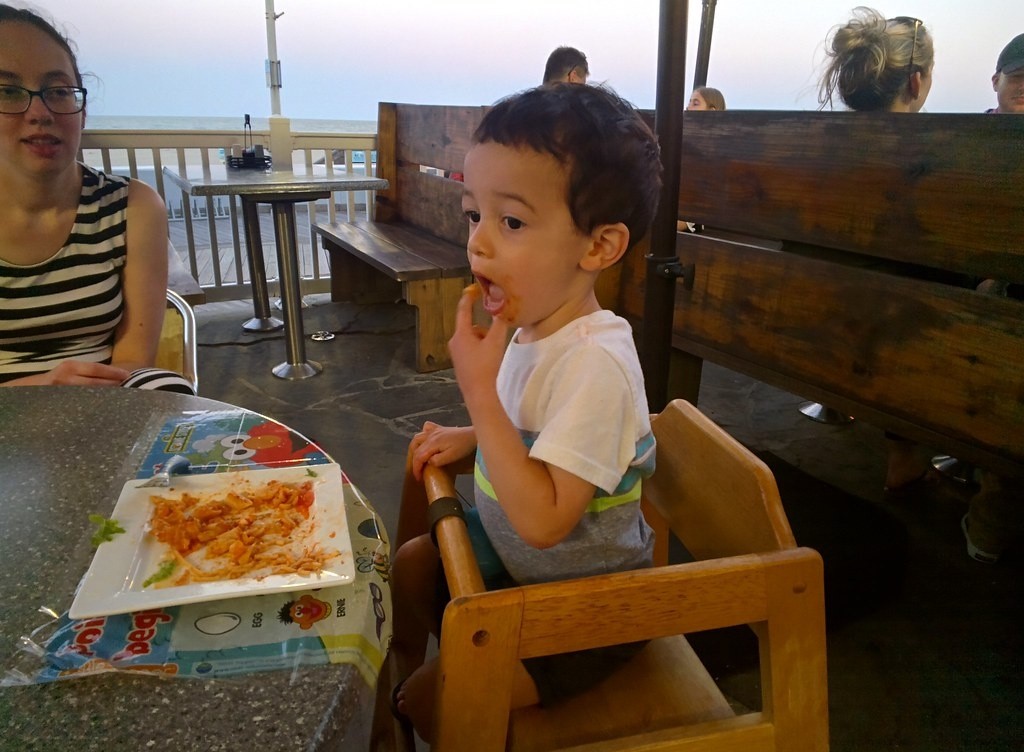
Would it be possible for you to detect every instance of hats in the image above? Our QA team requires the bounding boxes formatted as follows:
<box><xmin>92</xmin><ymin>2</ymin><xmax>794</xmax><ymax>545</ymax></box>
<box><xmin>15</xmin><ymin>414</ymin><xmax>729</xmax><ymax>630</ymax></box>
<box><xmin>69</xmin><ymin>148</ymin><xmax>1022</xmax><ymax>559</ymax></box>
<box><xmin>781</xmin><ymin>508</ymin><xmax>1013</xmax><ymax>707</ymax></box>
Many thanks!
<box><xmin>996</xmin><ymin>32</ymin><xmax>1024</xmax><ymax>75</ymax></box>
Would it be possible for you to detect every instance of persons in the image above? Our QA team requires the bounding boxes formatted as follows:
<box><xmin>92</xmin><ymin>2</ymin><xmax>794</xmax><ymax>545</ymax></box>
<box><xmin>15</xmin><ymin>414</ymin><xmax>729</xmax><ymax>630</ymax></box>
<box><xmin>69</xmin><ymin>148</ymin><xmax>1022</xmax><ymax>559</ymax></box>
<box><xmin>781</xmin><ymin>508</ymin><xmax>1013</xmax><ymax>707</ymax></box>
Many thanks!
<box><xmin>817</xmin><ymin>6</ymin><xmax>934</xmax><ymax>112</ymax></box>
<box><xmin>543</xmin><ymin>47</ymin><xmax>590</xmax><ymax>83</ymax></box>
<box><xmin>685</xmin><ymin>87</ymin><xmax>726</xmax><ymax>111</ymax></box>
<box><xmin>392</xmin><ymin>82</ymin><xmax>663</xmax><ymax>752</ymax></box>
<box><xmin>0</xmin><ymin>4</ymin><xmax>195</xmax><ymax>399</ymax></box>
<box><xmin>985</xmin><ymin>34</ymin><xmax>1024</xmax><ymax>114</ymax></box>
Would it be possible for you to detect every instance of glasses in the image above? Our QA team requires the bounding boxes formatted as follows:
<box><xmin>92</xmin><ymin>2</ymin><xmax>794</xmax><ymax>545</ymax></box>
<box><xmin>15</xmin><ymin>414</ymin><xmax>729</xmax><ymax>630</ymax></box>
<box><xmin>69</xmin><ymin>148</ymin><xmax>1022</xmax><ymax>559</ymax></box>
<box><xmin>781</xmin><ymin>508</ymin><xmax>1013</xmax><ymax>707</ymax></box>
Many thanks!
<box><xmin>0</xmin><ymin>83</ymin><xmax>88</xmax><ymax>115</ymax></box>
<box><xmin>886</xmin><ymin>15</ymin><xmax>923</xmax><ymax>93</ymax></box>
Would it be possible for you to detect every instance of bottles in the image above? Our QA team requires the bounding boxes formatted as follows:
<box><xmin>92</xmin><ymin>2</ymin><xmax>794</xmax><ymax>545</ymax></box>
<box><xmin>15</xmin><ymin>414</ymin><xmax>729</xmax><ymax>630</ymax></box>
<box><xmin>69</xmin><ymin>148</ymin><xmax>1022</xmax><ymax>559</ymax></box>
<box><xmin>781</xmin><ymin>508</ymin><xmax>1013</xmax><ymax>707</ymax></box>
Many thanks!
<box><xmin>233</xmin><ymin>143</ymin><xmax>243</xmax><ymax>165</ymax></box>
<box><xmin>254</xmin><ymin>145</ymin><xmax>265</xmax><ymax>167</ymax></box>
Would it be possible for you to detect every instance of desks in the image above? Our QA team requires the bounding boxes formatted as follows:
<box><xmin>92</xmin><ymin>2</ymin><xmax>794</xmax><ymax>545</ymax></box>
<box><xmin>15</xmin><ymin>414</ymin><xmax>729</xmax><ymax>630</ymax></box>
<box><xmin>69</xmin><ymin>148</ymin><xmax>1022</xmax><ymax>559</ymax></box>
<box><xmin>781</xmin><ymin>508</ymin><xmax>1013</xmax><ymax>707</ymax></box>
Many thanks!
<box><xmin>163</xmin><ymin>163</ymin><xmax>392</xmax><ymax>380</ymax></box>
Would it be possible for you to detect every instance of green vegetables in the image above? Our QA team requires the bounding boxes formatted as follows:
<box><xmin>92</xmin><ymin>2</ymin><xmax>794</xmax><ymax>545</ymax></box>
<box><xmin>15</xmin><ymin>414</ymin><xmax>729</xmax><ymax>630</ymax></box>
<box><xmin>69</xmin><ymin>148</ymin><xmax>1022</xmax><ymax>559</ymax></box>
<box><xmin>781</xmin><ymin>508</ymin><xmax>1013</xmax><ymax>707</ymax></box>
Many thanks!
<box><xmin>87</xmin><ymin>513</ymin><xmax>126</xmax><ymax>546</ymax></box>
<box><xmin>142</xmin><ymin>560</ymin><xmax>175</xmax><ymax>588</ymax></box>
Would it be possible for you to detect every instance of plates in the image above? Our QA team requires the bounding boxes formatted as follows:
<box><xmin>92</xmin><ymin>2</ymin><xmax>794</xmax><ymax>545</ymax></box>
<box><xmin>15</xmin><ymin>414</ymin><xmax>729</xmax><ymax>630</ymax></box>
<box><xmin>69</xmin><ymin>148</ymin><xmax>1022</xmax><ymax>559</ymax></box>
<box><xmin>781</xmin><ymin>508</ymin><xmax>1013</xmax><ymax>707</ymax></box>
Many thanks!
<box><xmin>68</xmin><ymin>462</ymin><xmax>356</xmax><ymax>620</ymax></box>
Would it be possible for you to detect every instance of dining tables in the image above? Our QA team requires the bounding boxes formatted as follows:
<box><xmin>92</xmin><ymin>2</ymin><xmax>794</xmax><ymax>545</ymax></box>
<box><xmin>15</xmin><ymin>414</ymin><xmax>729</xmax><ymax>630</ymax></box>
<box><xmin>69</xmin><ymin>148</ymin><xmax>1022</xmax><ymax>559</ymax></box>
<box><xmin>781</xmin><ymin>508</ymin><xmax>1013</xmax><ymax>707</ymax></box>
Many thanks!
<box><xmin>1</xmin><ymin>386</ymin><xmax>392</xmax><ymax>752</ymax></box>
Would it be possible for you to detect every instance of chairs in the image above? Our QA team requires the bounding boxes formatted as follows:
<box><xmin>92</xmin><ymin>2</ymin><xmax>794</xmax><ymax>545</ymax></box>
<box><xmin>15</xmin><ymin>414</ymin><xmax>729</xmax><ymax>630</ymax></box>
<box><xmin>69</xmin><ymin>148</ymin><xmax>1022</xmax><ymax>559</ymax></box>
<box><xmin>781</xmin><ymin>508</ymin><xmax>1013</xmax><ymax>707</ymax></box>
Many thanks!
<box><xmin>166</xmin><ymin>289</ymin><xmax>199</xmax><ymax>397</ymax></box>
<box><xmin>371</xmin><ymin>399</ymin><xmax>830</xmax><ymax>752</ymax></box>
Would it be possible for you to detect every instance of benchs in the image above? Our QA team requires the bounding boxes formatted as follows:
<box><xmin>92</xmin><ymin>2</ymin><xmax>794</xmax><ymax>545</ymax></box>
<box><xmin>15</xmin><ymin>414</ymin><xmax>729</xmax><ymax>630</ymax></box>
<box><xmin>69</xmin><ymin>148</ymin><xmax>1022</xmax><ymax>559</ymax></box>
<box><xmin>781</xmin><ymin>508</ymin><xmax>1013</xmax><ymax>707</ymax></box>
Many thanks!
<box><xmin>310</xmin><ymin>218</ymin><xmax>475</xmax><ymax>372</ymax></box>
<box><xmin>155</xmin><ymin>233</ymin><xmax>207</xmax><ymax>374</ymax></box>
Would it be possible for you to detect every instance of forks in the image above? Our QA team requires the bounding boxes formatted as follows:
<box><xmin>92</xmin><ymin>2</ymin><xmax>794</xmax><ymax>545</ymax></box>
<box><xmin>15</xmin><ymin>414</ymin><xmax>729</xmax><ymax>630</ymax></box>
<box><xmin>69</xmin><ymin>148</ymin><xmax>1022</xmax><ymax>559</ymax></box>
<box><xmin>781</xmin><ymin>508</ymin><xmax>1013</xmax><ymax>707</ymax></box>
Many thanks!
<box><xmin>134</xmin><ymin>457</ymin><xmax>188</xmax><ymax>488</ymax></box>
